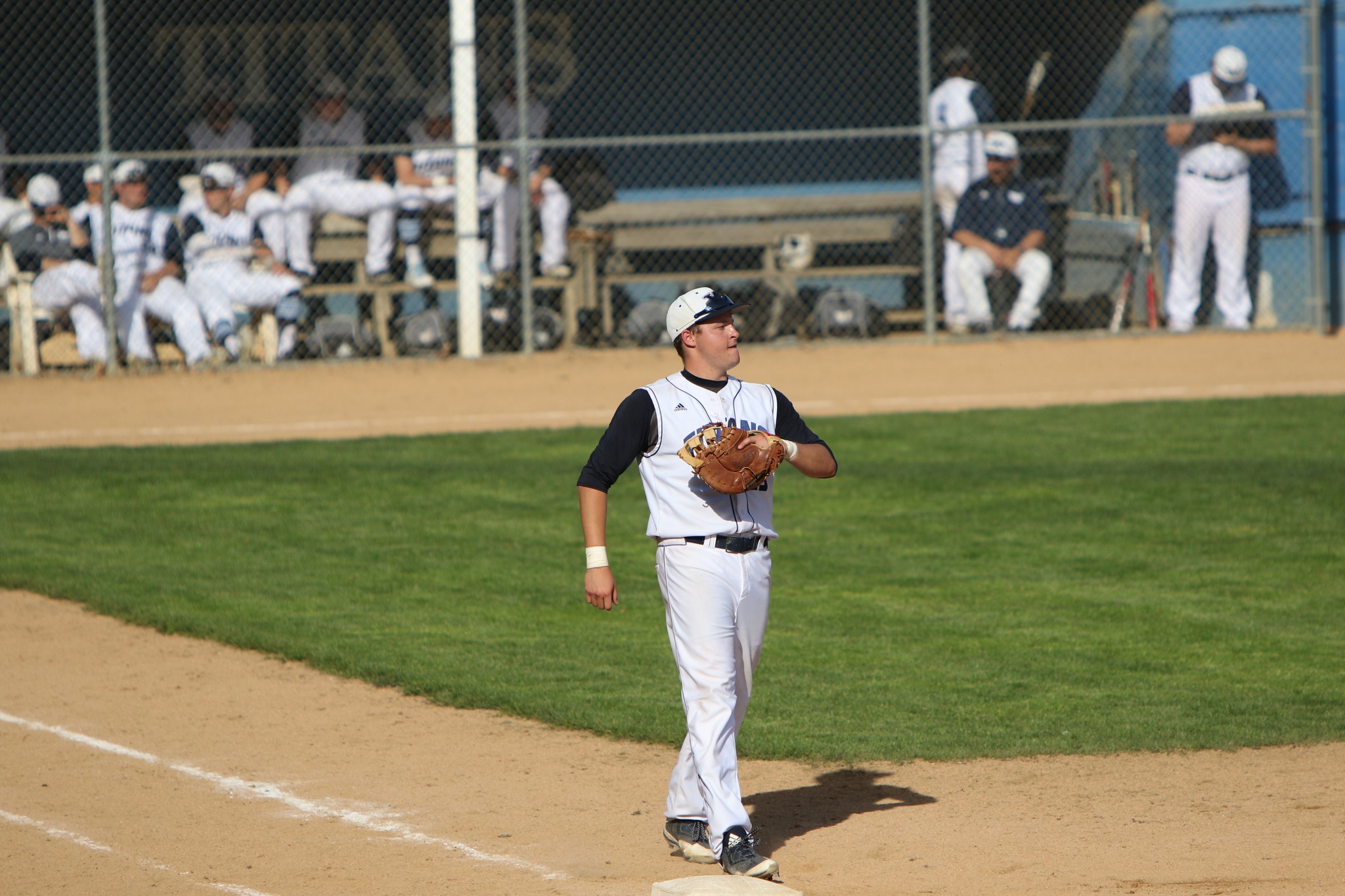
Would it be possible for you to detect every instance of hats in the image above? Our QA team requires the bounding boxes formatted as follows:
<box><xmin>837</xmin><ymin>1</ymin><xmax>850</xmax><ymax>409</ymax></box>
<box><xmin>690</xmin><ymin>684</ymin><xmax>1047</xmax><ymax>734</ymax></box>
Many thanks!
<box><xmin>26</xmin><ymin>174</ymin><xmax>59</xmax><ymax>206</ymax></box>
<box><xmin>943</xmin><ymin>48</ymin><xmax>980</xmax><ymax>72</ymax></box>
<box><xmin>313</xmin><ymin>75</ymin><xmax>347</xmax><ymax>99</ymax></box>
<box><xmin>423</xmin><ymin>97</ymin><xmax>450</xmax><ymax>122</ymax></box>
<box><xmin>112</xmin><ymin>159</ymin><xmax>150</xmax><ymax>183</ymax></box>
<box><xmin>1213</xmin><ymin>45</ymin><xmax>1246</xmax><ymax>83</ymax></box>
<box><xmin>203</xmin><ymin>161</ymin><xmax>236</xmax><ymax>188</ymax></box>
<box><xmin>85</xmin><ymin>163</ymin><xmax>102</xmax><ymax>183</ymax></box>
<box><xmin>665</xmin><ymin>286</ymin><xmax>750</xmax><ymax>342</ymax></box>
<box><xmin>984</xmin><ymin>133</ymin><xmax>1019</xmax><ymax>157</ymax></box>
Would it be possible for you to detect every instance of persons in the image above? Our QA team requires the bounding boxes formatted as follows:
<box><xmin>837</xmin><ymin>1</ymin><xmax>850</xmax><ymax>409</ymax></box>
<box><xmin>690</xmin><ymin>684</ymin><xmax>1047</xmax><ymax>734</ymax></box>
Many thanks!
<box><xmin>1166</xmin><ymin>45</ymin><xmax>1277</xmax><ymax>330</ymax></box>
<box><xmin>924</xmin><ymin>47</ymin><xmax>1000</xmax><ymax>334</ymax></box>
<box><xmin>574</xmin><ymin>288</ymin><xmax>839</xmax><ymax>878</ymax></box>
<box><xmin>0</xmin><ymin>54</ymin><xmax>574</xmax><ymax>371</ymax></box>
<box><xmin>284</xmin><ymin>71</ymin><xmax>396</xmax><ymax>285</ymax></box>
<box><xmin>944</xmin><ymin>134</ymin><xmax>1051</xmax><ymax>335</ymax></box>
<box><xmin>181</xmin><ymin>161</ymin><xmax>309</xmax><ymax>364</ymax></box>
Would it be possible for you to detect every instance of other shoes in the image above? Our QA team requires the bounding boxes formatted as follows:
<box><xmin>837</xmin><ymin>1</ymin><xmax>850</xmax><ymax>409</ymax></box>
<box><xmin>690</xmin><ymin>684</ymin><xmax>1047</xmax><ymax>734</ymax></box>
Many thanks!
<box><xmin>404</xmin><ymin>268</ymin><xmax>436</xmax><ymax>288</ymax></box>
<box><xmin>294</xmin><ymin>272</ymin><xmax>313</xmax><ymax>287</ymax></box>
<box><xmin>127</xmin><ymin>352</ymin><xmax>153</xmax><ymax>366</ymax></box>
<box><xmin>370</xmin><ymin>272</ymin><xmax>394</xmax><ymax>285</ymax></box>
<box><xmin>190</xmin><ymin>355</ymin><xmax>227</xmax><ymax>368</ymax></box>
<box><xmin>496</xmin><ymin>268</ymin><xmax>518</xmax><ymax>289</ymax></box>
<box><xmin>1167</xmin><ymin>316</ymin><xmax>1192</xmax><ymax>332</ymax></box>
<box><xmin>541</xmin><ymin>265</ymin><xmax>572</xmax><ymax>277</ymax></box>
<box><xmin>1226</xmin><ymin>317</ymin><xmax>1249</xmax><ymax>331</ymax></box>
<box><xmin>259</xmin><ymin>316</ymin><xmax>279</xmax><ymax>365</ymax></box>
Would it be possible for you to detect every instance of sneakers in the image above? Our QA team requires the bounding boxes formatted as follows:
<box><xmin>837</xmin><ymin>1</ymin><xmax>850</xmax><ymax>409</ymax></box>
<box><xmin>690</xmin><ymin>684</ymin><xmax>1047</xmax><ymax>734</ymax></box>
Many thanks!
<box><xmin>663</xmin><ymin>820</ymin><xmax>717</xmax><ymax>864</ymax></box>
<box><xmin>718</xmin><ymin>825</ymin><xmax>779</xmax><ymax>875</ymax></box>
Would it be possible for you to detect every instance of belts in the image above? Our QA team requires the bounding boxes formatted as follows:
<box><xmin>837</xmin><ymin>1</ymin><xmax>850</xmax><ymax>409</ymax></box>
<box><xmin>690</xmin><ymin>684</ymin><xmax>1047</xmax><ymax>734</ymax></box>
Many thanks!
<box><xmin>1188</xmin><ymin>169</ymin><xmax>1247</xmax><ymax>180</ymax></box>
<box><xmin>687</xmin><ymin>536</ymin><xmax>771</xmax><ymax>552</ymax></box>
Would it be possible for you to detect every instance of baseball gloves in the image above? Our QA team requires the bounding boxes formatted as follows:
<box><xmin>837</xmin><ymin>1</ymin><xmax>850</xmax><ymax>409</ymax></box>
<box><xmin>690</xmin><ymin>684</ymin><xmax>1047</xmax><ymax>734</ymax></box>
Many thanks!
<box><xmin>676</xmin><ymin>422</ymin><xmax>785</xmax><ymax>492</ymax></box>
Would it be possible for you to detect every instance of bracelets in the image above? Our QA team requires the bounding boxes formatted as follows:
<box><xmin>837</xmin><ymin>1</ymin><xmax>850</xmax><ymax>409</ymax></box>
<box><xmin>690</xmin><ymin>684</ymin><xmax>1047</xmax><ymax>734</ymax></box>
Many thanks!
<box><xmin>584</xmin><ymin>547</ymin><xmax>611</xmax><ymax>569</ymax></box>
<box><xmin>782</xmin><ymin>439</ymin><xmax>798</xmax><ymax>463</ymax></box>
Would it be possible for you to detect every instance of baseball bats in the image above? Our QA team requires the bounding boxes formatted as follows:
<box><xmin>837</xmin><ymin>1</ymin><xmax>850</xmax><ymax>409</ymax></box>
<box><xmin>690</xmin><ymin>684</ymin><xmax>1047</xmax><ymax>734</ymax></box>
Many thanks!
<box><xmin>1019</xmin><ymin>49</ymin><xmax>1054</xmax><ymax>121</ymax></box>
<box><xmin>1092</xmin><ymin>149</ymin><xmax>1157</xmax><ymax>334</ymax></box>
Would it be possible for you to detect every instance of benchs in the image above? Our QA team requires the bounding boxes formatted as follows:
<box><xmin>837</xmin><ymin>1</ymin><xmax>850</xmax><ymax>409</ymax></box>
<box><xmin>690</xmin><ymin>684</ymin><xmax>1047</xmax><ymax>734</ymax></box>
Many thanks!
<box><xmin>0</xmin><ymin>192</ymin><xmax>1071</xmax><ymax>373</ymax></box>
